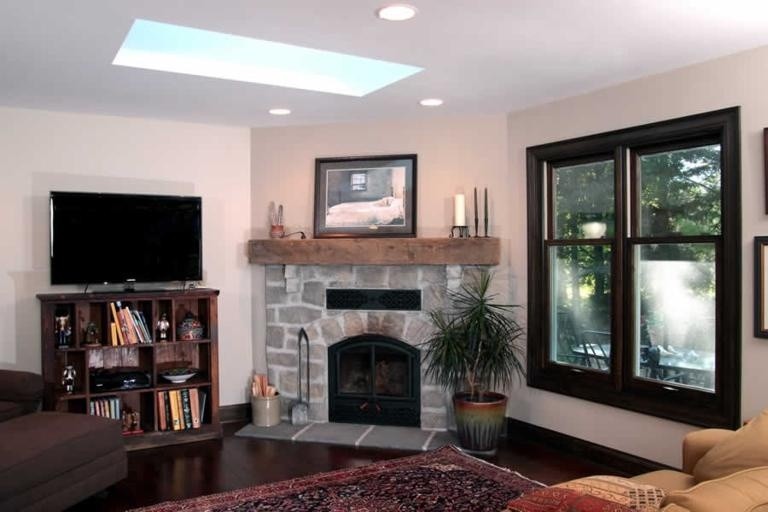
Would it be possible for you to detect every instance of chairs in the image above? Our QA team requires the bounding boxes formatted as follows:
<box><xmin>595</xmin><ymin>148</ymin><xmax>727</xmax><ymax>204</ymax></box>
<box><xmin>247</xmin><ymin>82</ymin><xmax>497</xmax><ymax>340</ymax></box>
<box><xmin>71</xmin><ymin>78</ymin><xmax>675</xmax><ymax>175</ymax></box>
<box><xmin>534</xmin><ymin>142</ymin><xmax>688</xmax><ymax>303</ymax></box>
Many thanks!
<box><xmin>647</xmin><ymin>346</ymin><xmax>684</xmax><ymax>382</ymax></box>
<box><xmin>0</xmin><ymin>369</ymin><xmax>127</xmax><ymax>512</ymax></box>
<box><xmin>581</xmin><ymin>331</ymin><xmax>610</xmax><ymax>373</ymax></box>
<box><xmin>502</xmin><ymin>428</ymin><xmax>768</xmax><ymax>512</ymax></box>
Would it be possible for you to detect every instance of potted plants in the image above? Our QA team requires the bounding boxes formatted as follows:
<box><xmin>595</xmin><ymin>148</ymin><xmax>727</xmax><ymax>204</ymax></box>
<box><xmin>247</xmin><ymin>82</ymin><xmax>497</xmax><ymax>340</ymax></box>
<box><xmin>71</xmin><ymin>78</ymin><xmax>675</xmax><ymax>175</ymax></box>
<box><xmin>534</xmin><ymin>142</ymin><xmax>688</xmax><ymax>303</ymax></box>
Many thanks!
<box><xmin>413</xmin><ymin>269</ymin><xmax>528</xmax><ymax>453</ymax></box>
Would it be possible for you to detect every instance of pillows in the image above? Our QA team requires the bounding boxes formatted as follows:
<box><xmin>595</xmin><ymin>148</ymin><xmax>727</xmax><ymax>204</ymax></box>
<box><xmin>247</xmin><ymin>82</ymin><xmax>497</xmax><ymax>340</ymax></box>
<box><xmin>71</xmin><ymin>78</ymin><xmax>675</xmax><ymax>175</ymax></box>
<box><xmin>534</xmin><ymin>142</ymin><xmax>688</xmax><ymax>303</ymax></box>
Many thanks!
<box><xmin>692</xmin><ymin>407</ymin><xmax>768</xmax><ymax>482</ymax></box>
<box><xmin>507</xmin><ymin>474</ymin><xmax>670</xmax><ymax>512</ymax></box>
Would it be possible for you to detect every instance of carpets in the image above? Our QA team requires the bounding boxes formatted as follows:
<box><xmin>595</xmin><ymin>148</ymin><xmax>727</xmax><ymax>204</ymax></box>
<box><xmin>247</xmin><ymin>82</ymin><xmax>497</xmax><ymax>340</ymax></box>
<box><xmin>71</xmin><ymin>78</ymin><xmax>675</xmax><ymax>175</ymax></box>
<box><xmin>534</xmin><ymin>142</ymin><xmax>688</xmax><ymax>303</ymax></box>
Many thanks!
<box><xmin>128</xmin><ymin>443</ymin><xmax>549</xmax><ymax>512</ymax></box>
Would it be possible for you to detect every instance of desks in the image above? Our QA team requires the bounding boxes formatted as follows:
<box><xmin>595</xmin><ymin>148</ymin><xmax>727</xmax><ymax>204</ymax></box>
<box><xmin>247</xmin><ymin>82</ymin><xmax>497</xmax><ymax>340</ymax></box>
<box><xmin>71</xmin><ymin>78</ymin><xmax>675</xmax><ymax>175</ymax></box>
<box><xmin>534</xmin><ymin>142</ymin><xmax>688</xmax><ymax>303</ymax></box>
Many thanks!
<box><xmin>572</xmin><ymin>344</ymin><xmax>715</xmax><ymax>391</ymax></box>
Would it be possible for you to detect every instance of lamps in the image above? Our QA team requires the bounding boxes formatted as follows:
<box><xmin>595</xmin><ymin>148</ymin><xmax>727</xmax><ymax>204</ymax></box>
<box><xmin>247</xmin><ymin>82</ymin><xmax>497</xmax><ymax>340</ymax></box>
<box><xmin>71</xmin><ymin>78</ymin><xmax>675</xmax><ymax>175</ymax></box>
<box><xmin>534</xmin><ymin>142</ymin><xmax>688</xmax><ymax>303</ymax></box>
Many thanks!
<box><xmin>577</xmin><ymin>214</ymin><xmax>607</xmax><ymax>238</ymax></box>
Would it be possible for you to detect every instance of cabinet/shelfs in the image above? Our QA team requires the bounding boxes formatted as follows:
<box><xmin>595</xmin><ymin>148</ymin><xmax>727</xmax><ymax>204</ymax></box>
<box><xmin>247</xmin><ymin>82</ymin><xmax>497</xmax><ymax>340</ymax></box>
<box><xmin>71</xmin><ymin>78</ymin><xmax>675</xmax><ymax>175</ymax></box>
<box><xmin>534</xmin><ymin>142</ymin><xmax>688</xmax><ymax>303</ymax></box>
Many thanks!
<box><xmin>35</xmin><ymin>288</ymin><xmax>223</xmax><ymax>452</ymax></box>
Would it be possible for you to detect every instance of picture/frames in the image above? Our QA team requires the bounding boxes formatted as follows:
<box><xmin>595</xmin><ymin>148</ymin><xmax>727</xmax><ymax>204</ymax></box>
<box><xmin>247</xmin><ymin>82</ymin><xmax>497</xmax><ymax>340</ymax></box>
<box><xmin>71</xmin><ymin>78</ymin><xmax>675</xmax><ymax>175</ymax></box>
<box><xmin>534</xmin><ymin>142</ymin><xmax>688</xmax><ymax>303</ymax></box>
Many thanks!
<box><xmin>314</xmin><ymin>153</ymin><xmax>417</xmax><ymax>238</ymax></box>
<box><xmin>762</xmin><ymin>127</ymin><xmax>768</xmax><ymax>214</ymax></box>
<box><xmin>753</xmin><ymin>236</ymin><xmax>768</xmax><ymax>338</ymax></box>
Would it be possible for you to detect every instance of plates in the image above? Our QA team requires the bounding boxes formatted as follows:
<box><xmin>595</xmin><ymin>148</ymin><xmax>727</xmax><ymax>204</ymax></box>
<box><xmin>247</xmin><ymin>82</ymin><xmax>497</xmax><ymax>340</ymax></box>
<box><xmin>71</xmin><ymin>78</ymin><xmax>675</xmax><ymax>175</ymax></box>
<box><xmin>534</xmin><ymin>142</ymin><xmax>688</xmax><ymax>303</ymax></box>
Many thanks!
<box><xmin>161</xmin><ymin>366</ymin><xmax>199</xmax><ymax>383</ymax></box>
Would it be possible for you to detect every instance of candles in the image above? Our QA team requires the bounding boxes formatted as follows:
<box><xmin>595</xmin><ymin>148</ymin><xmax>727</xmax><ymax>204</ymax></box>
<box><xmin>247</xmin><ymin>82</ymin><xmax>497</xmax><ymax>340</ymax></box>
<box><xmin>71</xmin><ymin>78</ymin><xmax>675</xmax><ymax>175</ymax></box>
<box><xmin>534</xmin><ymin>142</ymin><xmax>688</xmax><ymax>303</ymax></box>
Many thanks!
<box><xmin>454</xmin><ymin>194</ymin><xmax>466</xmax><ymax>226</ymax></box>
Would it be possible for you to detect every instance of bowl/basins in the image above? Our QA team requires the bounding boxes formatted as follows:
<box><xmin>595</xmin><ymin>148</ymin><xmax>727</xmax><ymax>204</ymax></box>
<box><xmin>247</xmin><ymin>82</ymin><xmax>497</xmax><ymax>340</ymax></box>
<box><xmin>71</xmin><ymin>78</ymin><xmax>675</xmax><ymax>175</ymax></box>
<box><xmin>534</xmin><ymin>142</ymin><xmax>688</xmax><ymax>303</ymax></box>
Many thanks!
<box><xmin>583</xmin><ymin>221</ymin><xmax>609</xmax><ymax>238</ymax></box>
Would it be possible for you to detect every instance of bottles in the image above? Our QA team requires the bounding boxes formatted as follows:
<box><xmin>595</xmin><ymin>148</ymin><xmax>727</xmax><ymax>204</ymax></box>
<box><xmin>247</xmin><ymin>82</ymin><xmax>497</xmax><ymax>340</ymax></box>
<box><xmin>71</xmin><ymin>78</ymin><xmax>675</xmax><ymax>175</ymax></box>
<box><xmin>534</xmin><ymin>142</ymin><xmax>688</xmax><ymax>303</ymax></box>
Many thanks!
<box><xmin>176</xmin><ymin>316</ymin><xmax>205</xmax><ymax>340</ymax></box>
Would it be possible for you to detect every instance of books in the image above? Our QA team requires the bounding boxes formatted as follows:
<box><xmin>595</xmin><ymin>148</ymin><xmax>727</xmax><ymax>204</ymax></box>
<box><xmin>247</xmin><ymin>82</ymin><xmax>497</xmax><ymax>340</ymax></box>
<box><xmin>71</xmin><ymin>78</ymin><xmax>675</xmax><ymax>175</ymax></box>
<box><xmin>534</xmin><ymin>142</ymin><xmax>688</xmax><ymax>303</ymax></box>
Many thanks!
<box><xmin>90</xmin><ymin>396</ymin><xmax>120</xmax><ymax>421</ymax></box>
<box><xmin>156</xmin><ymin>390</ymin><xmax>207</xmax><ymax>431</ymax></box>
<box><xmin>110</xmin><ymin>302</ymin><xmax>153</xmax><ymax>346</ymax></box>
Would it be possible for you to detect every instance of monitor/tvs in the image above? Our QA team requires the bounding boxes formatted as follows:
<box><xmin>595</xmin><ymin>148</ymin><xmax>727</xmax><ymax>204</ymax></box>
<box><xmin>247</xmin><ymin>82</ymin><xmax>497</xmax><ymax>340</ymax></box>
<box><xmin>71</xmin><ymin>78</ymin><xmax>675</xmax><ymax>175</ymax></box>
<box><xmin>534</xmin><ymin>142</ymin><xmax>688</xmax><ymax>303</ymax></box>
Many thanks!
<box><xmin>48</xmin><ymin>190</ymin><xmax>203</xmax><ymax>295</ymax></box>
